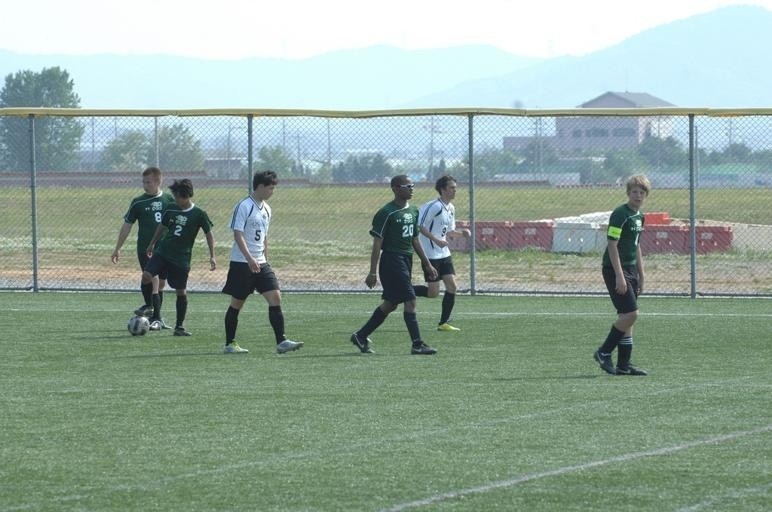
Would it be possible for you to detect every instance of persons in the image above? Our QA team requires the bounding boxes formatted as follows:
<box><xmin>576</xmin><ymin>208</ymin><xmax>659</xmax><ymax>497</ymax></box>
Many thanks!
<box><xmin>221</xmin><ymin>169</ymin><xmax>305</xmax><ymax>355</ymax></box>
<box><xmin>110</xmin><ymin>166</ymin><xmax>176</xmax><ymax>329</ymax></box>
<box><xmin>349</xmin><ymin>175</ymin><xmax>438</xmax><ymax>355</ymax></box>
<box><xmin>413</xmin><ymin>174</ymin><xmax>472</xmax><ymax>332</ymax></box>
<box><xmin>593</xmin><ymin>174</ymin><xmax>651</xmax><ymax>376</ymax></box>
<box><xmin>133</xmin><ymin>178</ymin><xmax>217</xmax><ymax>337</ymax></box>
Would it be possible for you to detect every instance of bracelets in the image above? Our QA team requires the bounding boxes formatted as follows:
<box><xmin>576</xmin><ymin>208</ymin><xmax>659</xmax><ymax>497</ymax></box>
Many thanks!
<box><xmin>369</xmin><ymin>273</ymin><xmax>376</xmax><ymax>277</ymax></box>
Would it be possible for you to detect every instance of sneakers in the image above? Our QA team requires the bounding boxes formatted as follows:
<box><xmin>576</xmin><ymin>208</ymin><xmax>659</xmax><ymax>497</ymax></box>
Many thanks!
<box><xmin>616</xmin><ymin>364</ymin><xmax>648</xmax><ymax>376</ymax></box>
<box><xmin>134</xmin><ymin>305</ymin><xmax>192</xmax><ymax>336</ymax></box>
<box><xmin>438</xmin><ymin>321</ymin><xmax>460</xmax><ymax>330</ymax></box>
<box><xmin>351</xmin><ymin>330</ymin><xmax>376</xmax><ymax>353</ymax></box>
<box><xmin>276</xmin><ymin>339</ymin><xmax>304</xmax><ymax>354</ymax></box>
<box><xmin>223</xmin><ymin>339</ymin><xmax>249</xmax><ymax>353</ymax></box>
<box><xmin>411</xmin><ymin>338</ymin><xmax>437</xmax><ymax>355</ymax></box>
<box><xmin>594</xmin><ymin>348</ymin><xmax>616</xmax><ymax>374</ymax></box>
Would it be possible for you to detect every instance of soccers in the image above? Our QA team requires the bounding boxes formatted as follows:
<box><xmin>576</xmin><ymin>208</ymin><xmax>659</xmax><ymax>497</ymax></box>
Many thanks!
<box><xmin>127</xmin><ymin>316</ymin><xmax>149</xmax><ymax>334</ymax></box>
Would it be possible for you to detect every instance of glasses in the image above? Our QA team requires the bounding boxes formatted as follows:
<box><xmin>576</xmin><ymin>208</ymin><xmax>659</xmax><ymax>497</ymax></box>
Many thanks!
<box><xmin>397</xmin><ymin>184</ymin><xmax>415</xmax><ymax>189</ymax></box>
